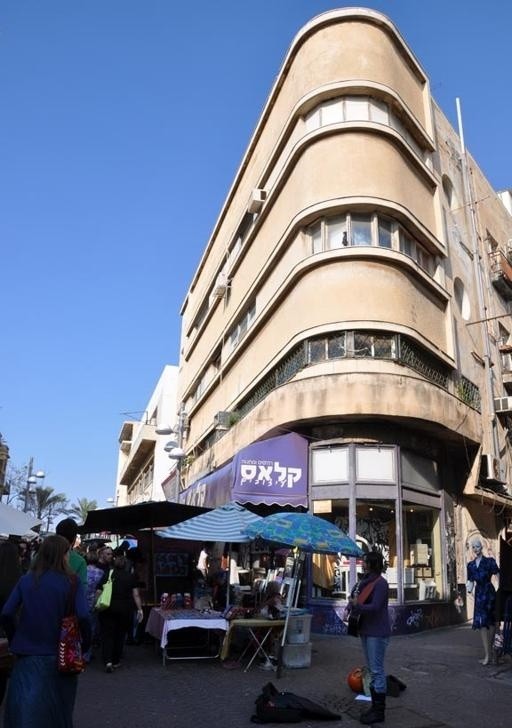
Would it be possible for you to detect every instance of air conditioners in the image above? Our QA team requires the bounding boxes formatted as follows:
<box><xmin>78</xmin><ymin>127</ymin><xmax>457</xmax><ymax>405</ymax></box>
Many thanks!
<box><xmin>212</xmin><ymin>274</ymin><xmax>229</xmax><ymax>296</ymax></box>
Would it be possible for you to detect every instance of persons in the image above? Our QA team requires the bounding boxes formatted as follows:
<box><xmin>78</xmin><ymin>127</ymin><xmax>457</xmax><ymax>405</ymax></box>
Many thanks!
<box><xmin>223</xmin><ymin>582</ymin><xmax>285</xmax><ymax>670</ymax></box>
<box><xmin>467</xmin><ymin>542</ymin><xmax>500</xmax><ymax>665</ymax></box>
<box><xmin>215</xmin><ymin>572</ymin><xmax>236</xmax><ymax>611</ymax></box>
<box><xmin>343</xmin><ymin>552</ymin><xmax>391</xmax><ymax>724</ymax></box>
<box><xmin>0</xmin><ymin>500</ymin><xmax>143</xmax><ymax>728</ymax></box>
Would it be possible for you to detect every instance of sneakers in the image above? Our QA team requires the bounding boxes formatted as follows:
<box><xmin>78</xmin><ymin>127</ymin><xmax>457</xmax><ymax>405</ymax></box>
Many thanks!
<box><xmin>222</xmin><ymin>661</ymin><xmax>240</xmax><ymax>669</ymax></box>
<box><xmin>104</xmin><ymin>663</ymin><xmax>112</xmax><ymax>673</ymax></box>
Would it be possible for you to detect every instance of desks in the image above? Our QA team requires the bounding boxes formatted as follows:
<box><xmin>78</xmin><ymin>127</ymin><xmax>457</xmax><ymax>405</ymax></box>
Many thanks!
<box><xmin>232</xmin><ymin>618</ymin><xmax>284</xmax><ymax>672</ymax></box>
<box><xmin>150</xmin><ymin>607</ymin><xmax>229</xmax><ymax>666</ymax></box>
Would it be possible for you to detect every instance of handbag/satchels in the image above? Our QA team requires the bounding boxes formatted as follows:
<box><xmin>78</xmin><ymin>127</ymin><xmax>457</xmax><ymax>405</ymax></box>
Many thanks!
<box><xmin>93</xmin><ymin>568</ymin><xmax>115</xmax><ymax>611</ymax></box>
<box><xmin>57</xmin><ymin>575</ymin><xmax>87</xmax><ymax>675</ymax></box>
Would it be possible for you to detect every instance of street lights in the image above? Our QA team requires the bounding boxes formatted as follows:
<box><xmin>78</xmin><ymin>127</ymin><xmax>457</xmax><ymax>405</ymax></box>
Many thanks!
<box><xmin>24</xmin><ymin>469</ymin><xmax>46</xmax><ymax>512</ymax></box>
<box><xmin>154</xmin><ymin>408</ymin><xmax>192</xmax><ymax>504</ymax></box>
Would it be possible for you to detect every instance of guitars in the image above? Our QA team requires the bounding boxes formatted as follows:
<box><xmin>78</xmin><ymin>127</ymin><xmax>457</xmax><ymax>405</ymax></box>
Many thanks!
<box><xmin>344</xmin><ymin>597</ymin><xmax>362</xmax><ymax>637</ymax></box>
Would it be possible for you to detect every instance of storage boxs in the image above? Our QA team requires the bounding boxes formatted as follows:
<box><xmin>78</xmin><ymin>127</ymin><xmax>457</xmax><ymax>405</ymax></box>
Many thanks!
<box><xmin>288</xmin><ymin>614</ymin><xmax>313</xmax><ymax>643</ymax></box>
<box><xmin>283</xmin><ymin>644</ymin><xmax>312</xmax><ymax>668</ymax></box>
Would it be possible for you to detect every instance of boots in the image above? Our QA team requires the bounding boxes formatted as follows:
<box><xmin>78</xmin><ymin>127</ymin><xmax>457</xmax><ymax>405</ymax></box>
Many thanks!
<box><xmin>361</xmin><ymin>687</ymin><xmax>374</xmax><ymax>716</ymax></box>
<box><xmin>360</xmin><ymin>692</ymin><xmax>385</xmax><ymax>723</ymax></box>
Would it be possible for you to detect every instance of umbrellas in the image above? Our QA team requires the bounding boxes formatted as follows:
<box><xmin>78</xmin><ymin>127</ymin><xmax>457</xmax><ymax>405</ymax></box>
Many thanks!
<box><xmin>79</xmin><ymin>500</ymin><xmax>215</xmax><ymax>606</ymax></box>
<box><xmin>154</xmin><ymin>501</ymin><xmax>264</xmax><ymax>608</ymax></box>
<box><xmin>240</xmin><ymin>510</ymin><xmax>364</xmax><ymax>647</ymax></box>
